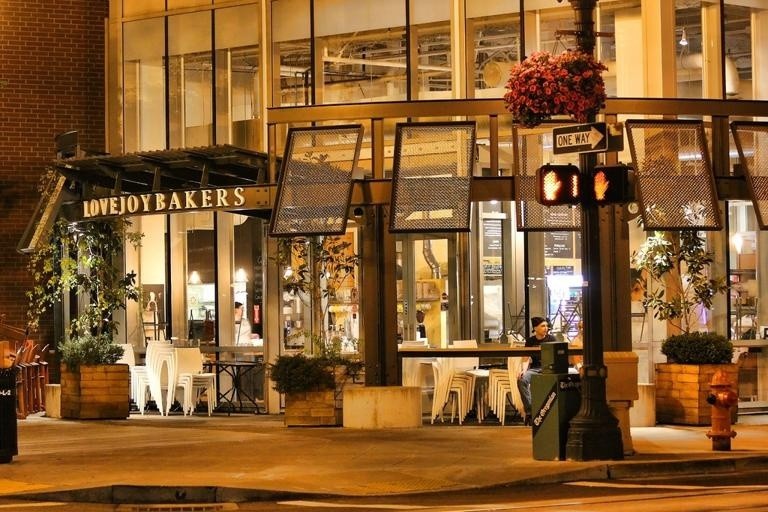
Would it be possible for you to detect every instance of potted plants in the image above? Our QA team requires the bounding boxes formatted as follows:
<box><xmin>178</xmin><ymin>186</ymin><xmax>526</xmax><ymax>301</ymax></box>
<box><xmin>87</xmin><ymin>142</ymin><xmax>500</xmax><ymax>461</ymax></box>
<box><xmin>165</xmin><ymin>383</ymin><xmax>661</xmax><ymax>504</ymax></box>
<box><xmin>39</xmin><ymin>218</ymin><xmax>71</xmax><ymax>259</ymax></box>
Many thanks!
<box><xmin>265</xmin><ymin>349</ymin><xmax>364</xmax><ymax>427</ymax></box>
<box><xmin>56</xmin><ymin>331</ymin><xmax>131</xmax><ymax>421</ymax></box>
<box><xmin>654</xmin><ymin>332</ymin><xmax>742</xmax><ymax>428</ymax></box>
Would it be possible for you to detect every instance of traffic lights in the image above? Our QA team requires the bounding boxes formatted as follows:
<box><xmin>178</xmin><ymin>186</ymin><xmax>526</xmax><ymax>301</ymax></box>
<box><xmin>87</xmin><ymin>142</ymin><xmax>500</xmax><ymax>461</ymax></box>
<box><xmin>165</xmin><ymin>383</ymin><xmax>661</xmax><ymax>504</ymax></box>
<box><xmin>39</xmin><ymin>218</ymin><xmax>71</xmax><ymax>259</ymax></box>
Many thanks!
<box><xmin>536</xmin><ymin>164</ymin><xmax>635</xmax><ymax>205</ymax></box>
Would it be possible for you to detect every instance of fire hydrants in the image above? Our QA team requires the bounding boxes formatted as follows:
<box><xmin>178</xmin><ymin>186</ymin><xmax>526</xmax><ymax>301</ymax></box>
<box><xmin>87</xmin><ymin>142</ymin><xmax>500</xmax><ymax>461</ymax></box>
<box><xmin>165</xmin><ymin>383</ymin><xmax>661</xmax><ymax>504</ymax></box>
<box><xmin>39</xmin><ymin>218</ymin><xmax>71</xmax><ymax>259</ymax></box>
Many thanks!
<box><xmin>705</xmin><ymin>368</ymin><xmax>737</xmax><ymax>450</ymax></box>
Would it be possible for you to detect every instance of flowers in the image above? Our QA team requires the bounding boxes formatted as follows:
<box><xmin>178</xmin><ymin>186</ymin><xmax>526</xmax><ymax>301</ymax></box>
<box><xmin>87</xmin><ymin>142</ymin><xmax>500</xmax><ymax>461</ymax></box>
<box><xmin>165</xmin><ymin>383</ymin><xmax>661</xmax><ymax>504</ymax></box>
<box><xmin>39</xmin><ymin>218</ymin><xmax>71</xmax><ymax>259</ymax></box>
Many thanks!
<box><xmin>503</xmin><ymin>47</ymin><xmax>611</xmax><ymax>125</ymax></box>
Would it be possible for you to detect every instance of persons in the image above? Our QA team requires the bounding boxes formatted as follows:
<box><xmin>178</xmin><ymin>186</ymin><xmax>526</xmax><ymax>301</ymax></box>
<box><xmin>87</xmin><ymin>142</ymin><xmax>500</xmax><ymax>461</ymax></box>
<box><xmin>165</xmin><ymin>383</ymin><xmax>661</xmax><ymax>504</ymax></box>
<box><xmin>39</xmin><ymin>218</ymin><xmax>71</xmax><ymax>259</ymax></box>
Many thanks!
<box><xmin>234</xmin><ymin>302</ymin><xmax>260</xmax><ymax>361</ymax></box>
<box><xmin>516</xmin><ymin>316</ymin><xmax>559</xmax><ymax>426</ymax></box>
<box><xmin>568</xmin><ymin>333</ymin><xmax>583</xmax><ymax>374</ymax></box>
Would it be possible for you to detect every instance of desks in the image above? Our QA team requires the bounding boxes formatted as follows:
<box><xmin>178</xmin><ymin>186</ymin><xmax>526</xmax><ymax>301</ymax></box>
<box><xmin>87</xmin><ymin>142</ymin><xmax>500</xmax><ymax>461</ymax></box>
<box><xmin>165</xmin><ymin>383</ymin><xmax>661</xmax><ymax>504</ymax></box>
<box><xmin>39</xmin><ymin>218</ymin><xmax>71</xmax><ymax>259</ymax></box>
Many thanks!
<box><xmin>201</xmin><ymin>360</ymin><xmax>265</xmax><ymax>418</ymax></box>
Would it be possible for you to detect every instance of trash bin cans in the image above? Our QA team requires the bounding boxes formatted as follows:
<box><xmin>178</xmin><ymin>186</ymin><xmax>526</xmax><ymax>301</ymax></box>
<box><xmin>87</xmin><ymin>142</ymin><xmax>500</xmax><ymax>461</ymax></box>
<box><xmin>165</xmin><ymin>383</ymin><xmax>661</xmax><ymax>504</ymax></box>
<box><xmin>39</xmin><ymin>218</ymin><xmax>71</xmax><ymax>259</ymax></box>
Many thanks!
<box><xmin>0</xmin><ymin>368</ymin><xmax>20</xmax><ymax>463</ymax></box>
<box><xmin>602</xmin><ymin>352</ymin><xmax>639</xmax><ymax>457</ymax></box>
<box><xmin>530</xmin><ymin>342</ymin><xmax>580</xmax><ymax>461</ymax></box>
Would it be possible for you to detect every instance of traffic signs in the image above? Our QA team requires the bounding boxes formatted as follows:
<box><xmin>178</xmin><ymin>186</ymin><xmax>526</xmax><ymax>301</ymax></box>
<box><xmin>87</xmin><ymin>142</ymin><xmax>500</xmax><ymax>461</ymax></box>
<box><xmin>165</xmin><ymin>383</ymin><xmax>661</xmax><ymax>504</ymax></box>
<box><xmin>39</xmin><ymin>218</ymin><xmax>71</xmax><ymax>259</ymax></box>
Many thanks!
<box><xmin>551</xmin><ymin>122</ymin><xmax>608</xmax><ymax>155</ymax></box>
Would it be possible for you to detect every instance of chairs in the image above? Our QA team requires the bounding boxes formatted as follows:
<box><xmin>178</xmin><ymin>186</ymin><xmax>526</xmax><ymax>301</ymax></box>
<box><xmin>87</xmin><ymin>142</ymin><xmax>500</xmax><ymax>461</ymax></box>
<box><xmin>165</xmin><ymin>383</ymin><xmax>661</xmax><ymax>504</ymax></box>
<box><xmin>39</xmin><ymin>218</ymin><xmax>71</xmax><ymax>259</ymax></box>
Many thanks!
<box><xmin>107</xmin><ymin>339</ymin><xmax>217</xmax><ymax>417</ymax></box>
<box><xmin>399</xmin><ymin>339</ymin><xmax>531</xmax><ymax>426</ymax></box>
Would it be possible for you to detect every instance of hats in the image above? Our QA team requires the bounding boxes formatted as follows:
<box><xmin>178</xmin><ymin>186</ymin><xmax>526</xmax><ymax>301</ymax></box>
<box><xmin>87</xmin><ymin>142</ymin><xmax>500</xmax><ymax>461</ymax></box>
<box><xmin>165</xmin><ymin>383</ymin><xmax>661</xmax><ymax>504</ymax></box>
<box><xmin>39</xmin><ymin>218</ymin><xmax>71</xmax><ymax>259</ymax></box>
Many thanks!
<box><xmin>531</xmin><ymin>315</ymin><xmax>544</xmax><ymax>331</ymax></box>
